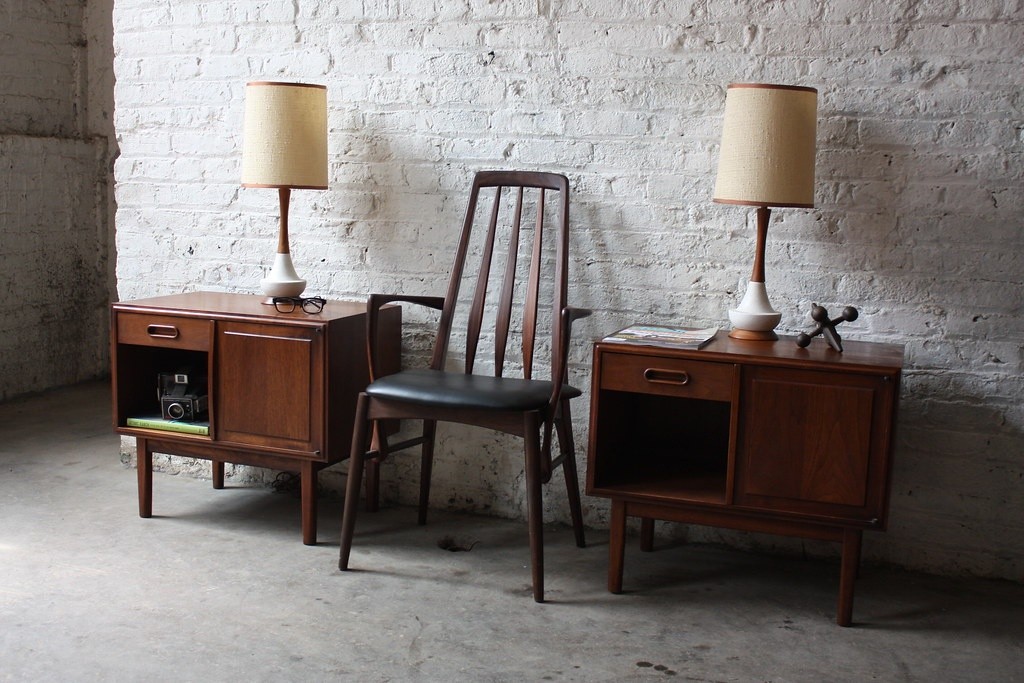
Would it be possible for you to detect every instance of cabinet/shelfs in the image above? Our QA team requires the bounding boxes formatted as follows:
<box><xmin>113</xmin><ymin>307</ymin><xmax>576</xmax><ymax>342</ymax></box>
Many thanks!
<box><xmin>584</xmin><ymin>324</ymin><xmax>905</xmax><ymax>631</ymax></box>
<box><xmin>108</xmin><ymin>291</ymin><xmax>403</xmax><ymax>547</ymax></box>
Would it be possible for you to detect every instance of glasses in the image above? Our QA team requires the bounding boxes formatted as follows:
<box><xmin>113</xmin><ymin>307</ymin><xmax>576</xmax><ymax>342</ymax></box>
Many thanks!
<box><xmin>273</xmin><ymin>296</ymin><xmax>326</xmax><ymax>314</ymax></box>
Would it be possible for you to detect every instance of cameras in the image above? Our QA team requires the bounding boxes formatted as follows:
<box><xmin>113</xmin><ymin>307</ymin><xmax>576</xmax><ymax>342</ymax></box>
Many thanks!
<box><xmin>158</xmin><ymin>372</ymin><xmax>208</xmax><ymax>422</ymax></box>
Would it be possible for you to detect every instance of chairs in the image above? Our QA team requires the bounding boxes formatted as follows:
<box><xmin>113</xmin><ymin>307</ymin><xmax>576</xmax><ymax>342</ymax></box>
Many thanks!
<box><xmin>338</xmin><ymin>170</ymin><xmax>592</xmax><ymax>603</ymax></box>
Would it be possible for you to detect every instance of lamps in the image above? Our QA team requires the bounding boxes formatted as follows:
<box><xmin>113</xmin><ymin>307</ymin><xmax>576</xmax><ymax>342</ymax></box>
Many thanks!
<box><xmin>239</xmin><ymin>80</ymin><xmax>329</xmax><ymax>305</ymax></box>
<box><xmin>712</xmin><ymin>84</ymin><xmax>819</xmax><ymax>340</ymax></box>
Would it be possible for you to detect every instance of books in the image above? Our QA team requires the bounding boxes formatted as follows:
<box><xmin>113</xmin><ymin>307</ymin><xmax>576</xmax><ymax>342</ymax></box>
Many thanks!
<box><xmin>601</xmin><ymin>322</ymin><xmax>720</xmax><ymax>351</ymax></box>
<box><xmin>127</xmin><ymin>409</ymin><xmax>209</xmax><ymax>436</ymax></box>
